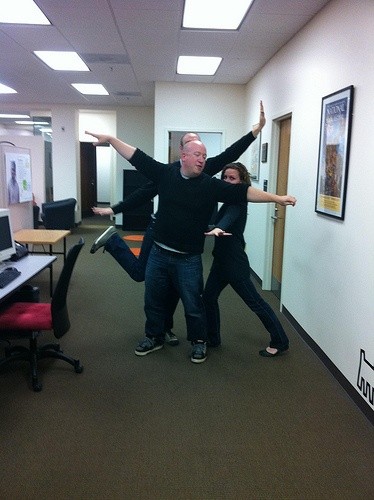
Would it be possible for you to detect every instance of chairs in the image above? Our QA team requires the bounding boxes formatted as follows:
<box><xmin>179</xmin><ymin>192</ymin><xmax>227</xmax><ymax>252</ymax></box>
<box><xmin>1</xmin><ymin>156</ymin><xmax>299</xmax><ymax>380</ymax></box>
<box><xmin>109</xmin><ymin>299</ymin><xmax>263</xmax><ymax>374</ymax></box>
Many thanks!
<box><xmin>0</xmin><ymin>236</ymin><xmax>84</xmax><ymax>394</ymax></box>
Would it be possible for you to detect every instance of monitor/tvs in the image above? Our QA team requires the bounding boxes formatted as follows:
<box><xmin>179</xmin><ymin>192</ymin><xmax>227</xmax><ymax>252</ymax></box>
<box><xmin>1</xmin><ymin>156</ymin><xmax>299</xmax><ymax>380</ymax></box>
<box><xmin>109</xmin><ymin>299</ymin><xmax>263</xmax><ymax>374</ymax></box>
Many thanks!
<box><xmin>0</xmin><ymin>208</ymin><xmax>17</xmax><ymax>262</ymax></box>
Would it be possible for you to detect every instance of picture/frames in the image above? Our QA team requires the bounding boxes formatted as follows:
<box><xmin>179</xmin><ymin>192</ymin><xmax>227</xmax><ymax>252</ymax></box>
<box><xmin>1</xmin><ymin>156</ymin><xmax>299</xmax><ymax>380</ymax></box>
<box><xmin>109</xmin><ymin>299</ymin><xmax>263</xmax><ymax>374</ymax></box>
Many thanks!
<box><xmin>315</xmin><ymin>84</ymin><xmax>355</xmax><ymax>220</ymax></box>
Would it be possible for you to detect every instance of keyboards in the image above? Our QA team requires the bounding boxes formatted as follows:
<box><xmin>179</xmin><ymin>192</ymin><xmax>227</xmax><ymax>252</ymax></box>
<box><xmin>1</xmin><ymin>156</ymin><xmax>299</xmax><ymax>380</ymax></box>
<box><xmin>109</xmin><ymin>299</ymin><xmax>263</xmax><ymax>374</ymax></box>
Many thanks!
<box><xmin>0</xmin><ymin>270</ymin><xmax>21</xmax><ymax>288</ymax></box>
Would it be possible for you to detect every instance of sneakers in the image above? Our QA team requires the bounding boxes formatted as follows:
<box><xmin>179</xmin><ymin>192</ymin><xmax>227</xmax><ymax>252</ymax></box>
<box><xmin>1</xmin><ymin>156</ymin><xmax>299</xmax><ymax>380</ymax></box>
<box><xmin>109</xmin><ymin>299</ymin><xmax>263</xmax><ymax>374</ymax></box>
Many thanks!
<box><xmin>90</xmin><ymin>226</ymin><xmax>117</xmax><ymax>254</ymax></box>
<box><xmin>135</xmin><ymin>336</ymin><xmax>164</xmax><ymax>355</ymax></box>
<box><xmin>190</xmin><ymin>339</ymin><xmax>208</xmax><ymax>363</ymax></box>
<box><xmin>165</xmin><ymin>329</ymin><xmax>179</xmax><ymax>346</ymax></box>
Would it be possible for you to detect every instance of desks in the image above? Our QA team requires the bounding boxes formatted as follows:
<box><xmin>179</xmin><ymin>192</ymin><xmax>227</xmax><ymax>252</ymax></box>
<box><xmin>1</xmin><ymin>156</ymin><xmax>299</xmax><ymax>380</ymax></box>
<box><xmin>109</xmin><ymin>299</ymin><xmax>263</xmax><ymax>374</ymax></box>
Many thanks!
<box><xmin>0</xmin><ymin>251</ymin><xmax>57</xmax><ymax>305</ymax></box>
<box><xmin>12</xmin><ymin>228</ymin><xmax>71</xmax><ymax>299</ymax></box>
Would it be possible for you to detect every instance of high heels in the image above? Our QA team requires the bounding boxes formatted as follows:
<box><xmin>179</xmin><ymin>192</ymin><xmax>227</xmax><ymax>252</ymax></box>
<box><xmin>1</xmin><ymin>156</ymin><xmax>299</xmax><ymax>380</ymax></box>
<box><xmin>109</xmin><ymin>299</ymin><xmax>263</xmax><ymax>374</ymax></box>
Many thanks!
<box><xmin>259</xmin><ymin>344</ymin><xmax>289</xmax><ymax>357</ymax></box>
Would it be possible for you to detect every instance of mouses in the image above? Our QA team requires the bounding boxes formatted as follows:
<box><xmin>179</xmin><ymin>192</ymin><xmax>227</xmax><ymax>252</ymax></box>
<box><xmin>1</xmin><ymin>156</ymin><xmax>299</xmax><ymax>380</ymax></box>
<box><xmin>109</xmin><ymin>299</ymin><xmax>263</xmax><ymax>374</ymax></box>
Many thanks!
<box><xmin>6</xmin><ymin>267</ymin><xmax>17</xmax><ymax>271</ymax></box>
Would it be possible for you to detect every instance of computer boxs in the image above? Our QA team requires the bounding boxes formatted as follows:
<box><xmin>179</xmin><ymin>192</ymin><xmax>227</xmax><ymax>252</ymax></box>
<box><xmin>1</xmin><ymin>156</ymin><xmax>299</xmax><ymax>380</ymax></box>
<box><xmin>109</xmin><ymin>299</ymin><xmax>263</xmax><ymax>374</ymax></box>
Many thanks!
<box><xmin>1</xmin><ymin>284</ymin><xmax>40</xmax><ymax>337</ymax></box>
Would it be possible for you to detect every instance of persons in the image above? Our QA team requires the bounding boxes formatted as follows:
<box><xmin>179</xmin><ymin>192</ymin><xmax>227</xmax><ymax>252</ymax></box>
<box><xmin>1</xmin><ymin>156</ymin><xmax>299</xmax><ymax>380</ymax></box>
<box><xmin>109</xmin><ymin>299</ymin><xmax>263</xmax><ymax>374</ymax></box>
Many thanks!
<box><xmin>86</xmin><ymin>131</ymin><xmax>296</xmax><ymax>364</ymax></box>
<box><xmin>204</xmin><ymin>162</ymin><xmax>290</xmax><ymax>356</ymax></box>
<box><xmin>89</xmin><ymin>100</ymin><xmax>266</xmax><ymax>346</ymax></box>
<box><xmin>8</xmin><ymin>161</ymin><xmax>19</xmax><ymax>204</ymax></box>
